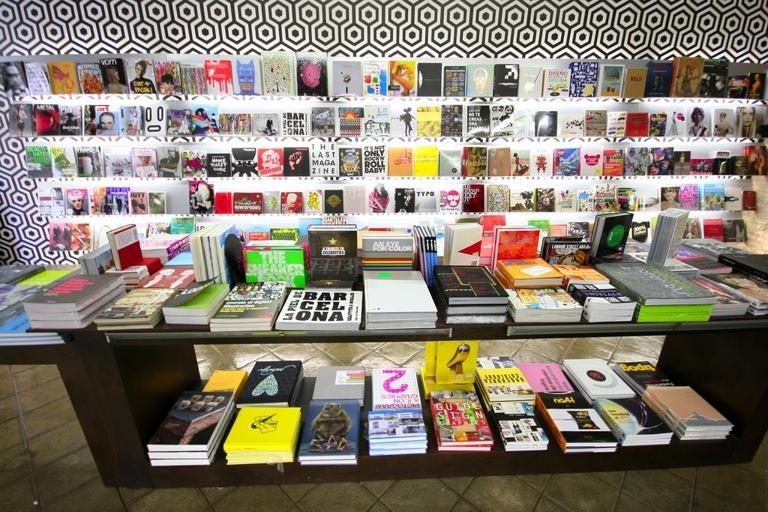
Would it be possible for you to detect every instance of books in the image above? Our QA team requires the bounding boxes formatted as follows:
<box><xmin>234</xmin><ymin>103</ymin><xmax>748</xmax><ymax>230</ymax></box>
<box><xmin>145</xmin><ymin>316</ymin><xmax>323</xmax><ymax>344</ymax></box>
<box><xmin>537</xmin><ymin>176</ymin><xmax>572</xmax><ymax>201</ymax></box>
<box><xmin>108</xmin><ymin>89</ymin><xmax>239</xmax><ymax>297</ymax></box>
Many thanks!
<box><xmin>146</xmin><ymin>355</ymin><xmax>733</xmax><ymax>466</ymax></box>
<box><xmin>0</xmin><ymin>50</ymin><xmax>768</xmax><ymax>346</ymax></box>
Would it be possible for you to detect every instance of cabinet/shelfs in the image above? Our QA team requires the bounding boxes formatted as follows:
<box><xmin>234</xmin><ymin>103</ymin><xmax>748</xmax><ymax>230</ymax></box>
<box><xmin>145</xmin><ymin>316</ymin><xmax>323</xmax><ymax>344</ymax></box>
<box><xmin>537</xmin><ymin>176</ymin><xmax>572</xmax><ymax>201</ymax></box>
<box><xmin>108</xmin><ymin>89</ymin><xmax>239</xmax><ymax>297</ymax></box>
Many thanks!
<box><xmin>0</xmin><ymin>52</ymin><xmax>768</xmax><ymax>256</ymax></box>
